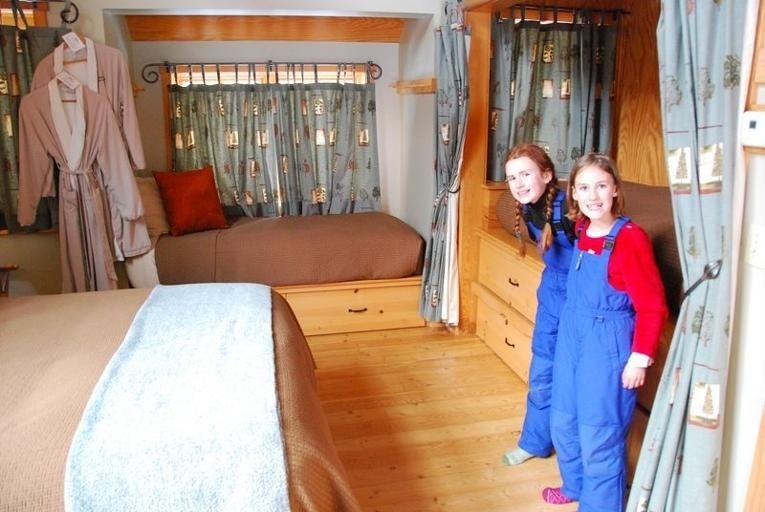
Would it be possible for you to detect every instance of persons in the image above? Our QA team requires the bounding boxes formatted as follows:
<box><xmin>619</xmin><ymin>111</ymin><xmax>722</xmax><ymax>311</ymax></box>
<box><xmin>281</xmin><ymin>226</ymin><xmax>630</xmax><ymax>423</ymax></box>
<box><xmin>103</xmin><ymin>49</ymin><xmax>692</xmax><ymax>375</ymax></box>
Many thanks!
<box><xmin>542</xmin><ymin>152</ymin><xmax>671</xmax><ymax>511</ymax></box>
<box><xmin>500</xmin><ymin>143</ymin><xmax>576</xmax><ymax>465</ymax></box>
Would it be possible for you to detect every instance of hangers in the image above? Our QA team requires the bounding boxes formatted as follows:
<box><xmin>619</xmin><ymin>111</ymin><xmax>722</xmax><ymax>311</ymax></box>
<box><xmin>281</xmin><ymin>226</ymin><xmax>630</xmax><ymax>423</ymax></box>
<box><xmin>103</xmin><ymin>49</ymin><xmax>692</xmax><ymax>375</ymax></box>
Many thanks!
<box><xmin>64</xmin><ymin>32</ymin><xmax>88</xmax><ymax>64</ymax></box>
<box><xmin>57</xmin><ymin>79</ymin><xmax>77</xmax><ymax>102</ymax></box>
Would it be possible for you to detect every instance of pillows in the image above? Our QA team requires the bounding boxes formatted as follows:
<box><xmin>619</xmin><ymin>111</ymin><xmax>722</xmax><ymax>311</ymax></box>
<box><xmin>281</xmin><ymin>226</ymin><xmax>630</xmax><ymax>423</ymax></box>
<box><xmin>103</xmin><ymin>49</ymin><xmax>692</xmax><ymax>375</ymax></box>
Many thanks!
<box><xmin>134</xmin><ymin>177</ymin><xmax>170</xmax><ymax>235</ymax></box>
<box><xmin>152</xmin><ymin>165</ymin><xmax>230</xmax><ymax>236</ymax></box>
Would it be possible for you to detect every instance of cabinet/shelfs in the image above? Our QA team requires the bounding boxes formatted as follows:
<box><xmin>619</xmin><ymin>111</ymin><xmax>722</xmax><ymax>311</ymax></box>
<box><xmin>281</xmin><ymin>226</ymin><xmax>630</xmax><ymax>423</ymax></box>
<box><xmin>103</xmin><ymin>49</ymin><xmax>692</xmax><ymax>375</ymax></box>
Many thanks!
<box><xmin>478</xmin><ymin>238</ymin><xmax>543</xmax><ymax>324</ymax></box>
<box><xmin>476</xmin><ymin>297</ymin><xmax>532</xmax><ymax>384</ymax></box>
<box><xmin>286</xmin><ymin>285</ymin><xmax>426</xmax><ymax>336</ymax></box>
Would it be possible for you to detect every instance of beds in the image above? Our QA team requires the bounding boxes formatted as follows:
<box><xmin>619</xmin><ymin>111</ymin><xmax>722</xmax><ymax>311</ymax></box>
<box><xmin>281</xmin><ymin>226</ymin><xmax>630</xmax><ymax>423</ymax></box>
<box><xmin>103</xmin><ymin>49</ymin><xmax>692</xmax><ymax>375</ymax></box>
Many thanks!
<box><xmin>154</xmin><ymin>215</ymin><xmax>426</xmax><ymax>335</ymax></box>
<box><xmin>0</xmin><ymin>282</ymin><xmax>362</xmax><ymax>512</ymax></box>
